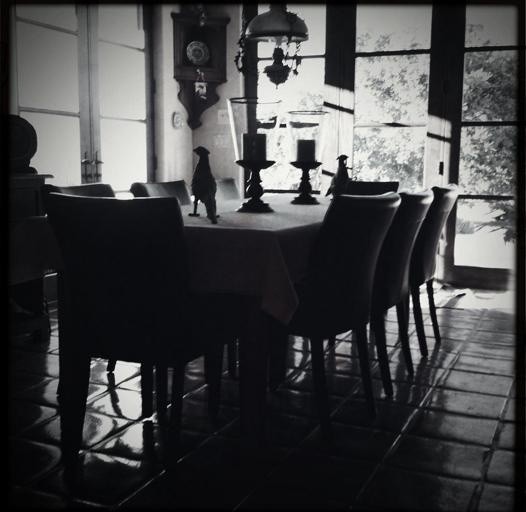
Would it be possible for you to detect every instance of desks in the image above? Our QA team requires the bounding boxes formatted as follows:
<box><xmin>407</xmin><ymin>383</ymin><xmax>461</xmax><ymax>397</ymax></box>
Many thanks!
<box><xmin>171</xmin><ymin>190</ymin><xmax>333</xmax><ymax>428</ymax></box>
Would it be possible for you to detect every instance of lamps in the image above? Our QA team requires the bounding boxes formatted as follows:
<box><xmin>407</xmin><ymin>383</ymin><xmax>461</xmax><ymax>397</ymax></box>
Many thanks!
<box><xmin>245</xmin><ymin>2</ymin><xmax>310</xmax><ymax>93</ymax></box>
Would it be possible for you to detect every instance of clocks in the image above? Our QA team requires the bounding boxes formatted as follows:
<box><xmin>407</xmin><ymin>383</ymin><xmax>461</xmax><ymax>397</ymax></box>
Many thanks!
<box><xmin>170</xmin><ymin>12</ymin><xmax>231</xmax><ymax>130</ymax></box>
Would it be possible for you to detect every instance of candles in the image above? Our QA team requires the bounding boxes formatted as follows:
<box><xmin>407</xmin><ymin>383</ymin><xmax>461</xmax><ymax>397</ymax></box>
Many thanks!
<box><xmin>297</xmin><ymin>138</ymin><xmax>316</xmax><ymax>161</ymax></box>
<box><xmin>242</xmin><ymin>133</ymin><xmax>266</xmax><ymax>161</ymax></box>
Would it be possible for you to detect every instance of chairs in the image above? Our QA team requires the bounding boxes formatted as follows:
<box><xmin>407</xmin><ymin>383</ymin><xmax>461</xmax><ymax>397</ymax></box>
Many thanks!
<box><xmin>410</xmin><ymin>183</ymin><xmax>458</xmax><ymax>358</ymax></box>
<box><xmin>49</xmin><ymin>192</ymin><xmax>234</xmax><ymax>470</ymax></box>
<box><xmin>45</xmin><ymin>184</ymin><xmax>116</xmax><ymax>197</ymax></box>
<box><xmin>372</xmin><ymin>188</ymin><xmax>434</xmax><ymax>398</ymax></box>
<box><xmin>280</xmin><ymin>191</ymin><xmax>402</xmax><ymax>440</ymax></box>
<box><xmin>129</xmin><ymin>179</ymin><xmax>191</xmax><ymax>206</ymax></box>
